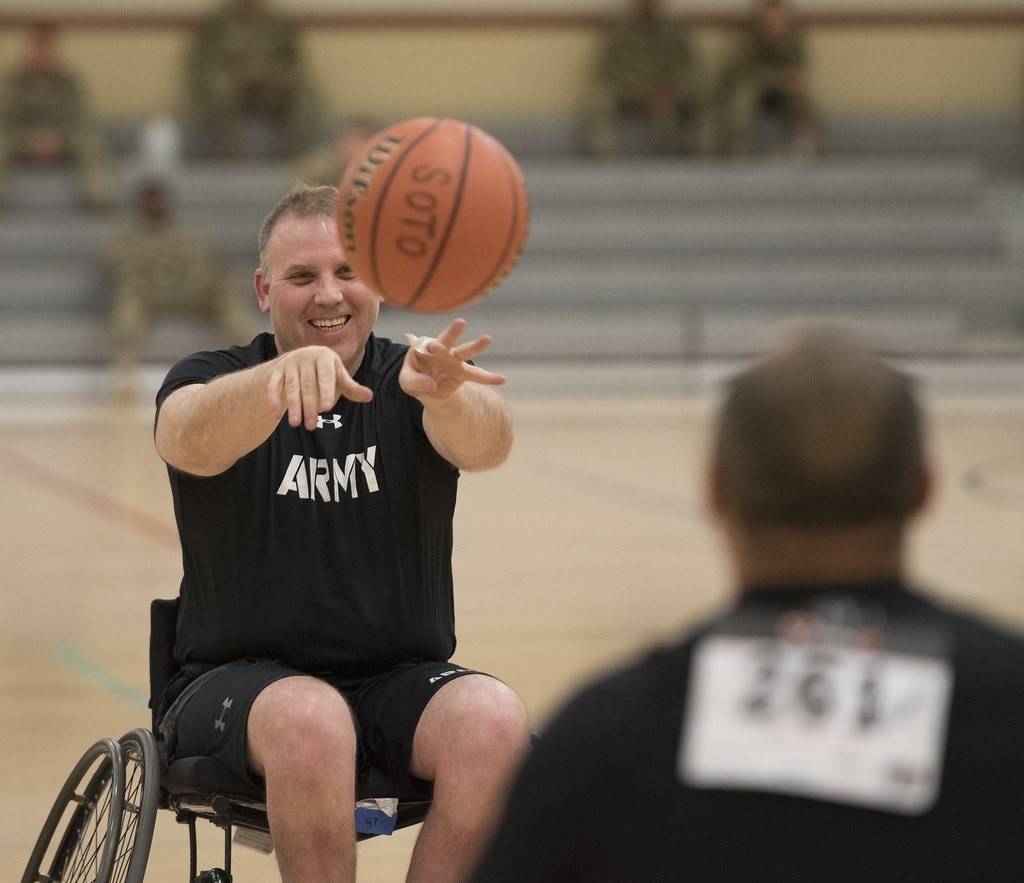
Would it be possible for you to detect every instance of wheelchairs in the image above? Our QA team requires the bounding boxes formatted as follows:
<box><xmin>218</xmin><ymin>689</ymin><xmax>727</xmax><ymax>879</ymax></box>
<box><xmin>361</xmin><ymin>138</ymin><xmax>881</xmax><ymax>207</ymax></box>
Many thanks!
<box><xmin>19</xmin><ymin>598</ymin><xmax>541</xmax><ymax>883</ymax></box>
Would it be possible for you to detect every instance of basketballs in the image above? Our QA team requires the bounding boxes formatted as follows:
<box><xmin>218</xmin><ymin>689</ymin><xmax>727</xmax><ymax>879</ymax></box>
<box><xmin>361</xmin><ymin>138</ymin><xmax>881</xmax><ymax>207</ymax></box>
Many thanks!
<box><xmin>337</xmin><ymin>116</ymin><xmax>529</xmax><ymax>317</ymax></box>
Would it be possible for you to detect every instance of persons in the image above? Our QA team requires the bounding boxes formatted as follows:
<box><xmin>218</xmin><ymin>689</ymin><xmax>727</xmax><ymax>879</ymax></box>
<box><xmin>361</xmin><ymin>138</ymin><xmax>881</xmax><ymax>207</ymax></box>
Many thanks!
<box><xmin>0</xmin><ymin>0</ymin><xmax>821</xmax><ymax>397</ymax></box>
<box><xmin>154</xmin><ymin>186</ymin><xmax>529</xmax><ymax>882</ymax></box>
<box><xmin>466</xmin><ymin>325</ymin><xmax>1024</xmax><ymax>883</ymax></box>
